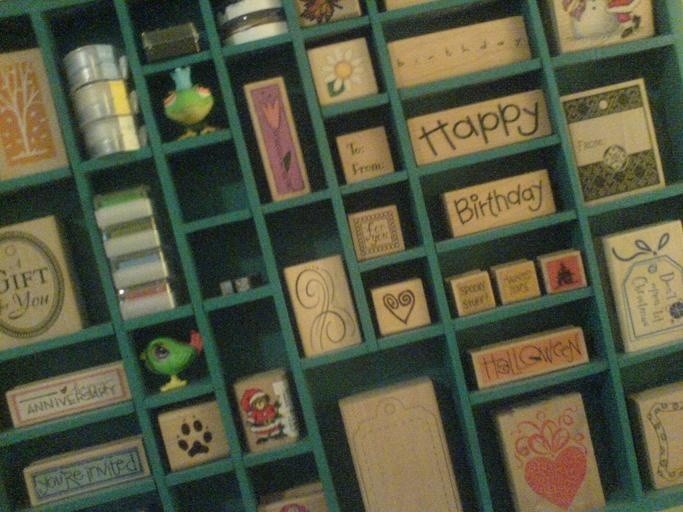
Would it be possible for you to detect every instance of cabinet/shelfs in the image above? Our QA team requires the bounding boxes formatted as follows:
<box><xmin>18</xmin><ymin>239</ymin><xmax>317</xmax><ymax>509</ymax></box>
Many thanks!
<box><xmin>0</xmin><ymin>0</ymin><xmax>683</xmax><ymax>512</ymax></box>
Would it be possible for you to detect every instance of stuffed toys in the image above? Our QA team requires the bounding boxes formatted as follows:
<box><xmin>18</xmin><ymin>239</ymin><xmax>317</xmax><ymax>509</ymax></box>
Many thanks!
<box><xmin>165</xmin><ymin>68</ymin><xmax>217</xmax><ymax>140</ymax></box>
<box><xmin>138</xmin><ymin>331</ymin><xmax>203</xmax><ymax>392</ymax></box>
